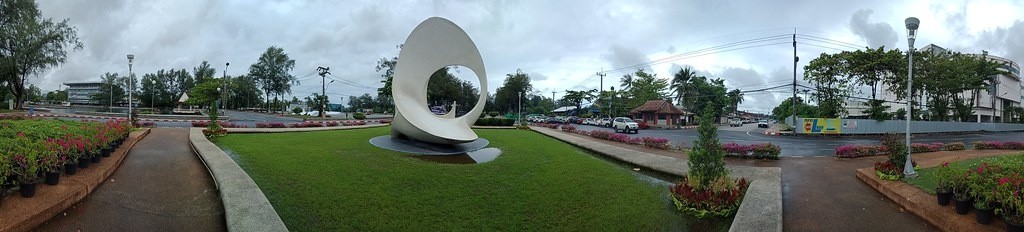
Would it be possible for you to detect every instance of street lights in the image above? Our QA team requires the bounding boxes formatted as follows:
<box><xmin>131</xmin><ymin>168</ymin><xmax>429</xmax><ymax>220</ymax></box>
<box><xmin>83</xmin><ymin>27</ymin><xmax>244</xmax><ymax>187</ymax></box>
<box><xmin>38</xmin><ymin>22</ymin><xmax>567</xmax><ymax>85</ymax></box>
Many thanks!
<box><xmin>126</xmin><ymin>54</ymin><xmax>135</xmax><ymax>124</ymax></box>
<box><xmin>518</xmin><ymin>87</ymin><xmax>522</xmax><ymax>128</ymax></box>
<box><xmin>323</xmin><ymin>80</ymin><xmax>334</xmax><ymax>120</ymax></box>
<box><xmin>222</xmin><ymin>62</ymin><xmax>230</xmax><ymax>117</ymax></box>
<box><xmin>902</xmin><ymin>16</ymin><xmax>922</xmax><ymax>178</ymax></box>
<box><xmin>151</xmin><ymin>79</ymin><xmax>157</xmax><ymax>114</ymax></box>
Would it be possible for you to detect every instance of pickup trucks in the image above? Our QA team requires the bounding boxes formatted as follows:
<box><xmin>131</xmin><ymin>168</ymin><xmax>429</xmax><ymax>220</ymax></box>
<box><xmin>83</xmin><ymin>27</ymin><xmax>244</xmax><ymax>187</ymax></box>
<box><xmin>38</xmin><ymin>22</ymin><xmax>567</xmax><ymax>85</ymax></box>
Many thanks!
<box><xmin>729</xmin><ymin>118</ymin><xmax>742</xmax><ymax>127</ymax></box>
<box><xmin>758</xmin><ymin>120</ymin><xmax>768</xmax><ymax>128</ymax></box>
<box><xmin>742</xmin><ymin>118</ymin><xmax>750</xmax><ymax>123</ymax></box>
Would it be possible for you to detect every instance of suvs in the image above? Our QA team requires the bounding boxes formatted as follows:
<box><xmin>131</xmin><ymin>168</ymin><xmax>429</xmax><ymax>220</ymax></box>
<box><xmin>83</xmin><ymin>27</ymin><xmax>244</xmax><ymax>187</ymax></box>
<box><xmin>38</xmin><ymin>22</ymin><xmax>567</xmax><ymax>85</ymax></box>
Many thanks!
<box><xmin>612</xmin><ymin>116</ymin><xmax>639</xmax><ymax>134</ymax></box>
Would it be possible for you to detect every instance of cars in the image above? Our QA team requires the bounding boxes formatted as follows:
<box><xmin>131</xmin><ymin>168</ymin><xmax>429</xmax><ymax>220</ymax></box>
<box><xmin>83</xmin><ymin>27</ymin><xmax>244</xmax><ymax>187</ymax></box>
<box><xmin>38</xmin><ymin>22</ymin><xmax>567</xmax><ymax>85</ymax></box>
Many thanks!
<box><xmin>526</xmin><ymin>114</ymin><xmax>612</xmax><ymax>128</ymax></box>
<box><xmin>634</xmin><ymin>118</ymin><xmax>650</xmax><ymax>130</ymax></box>
